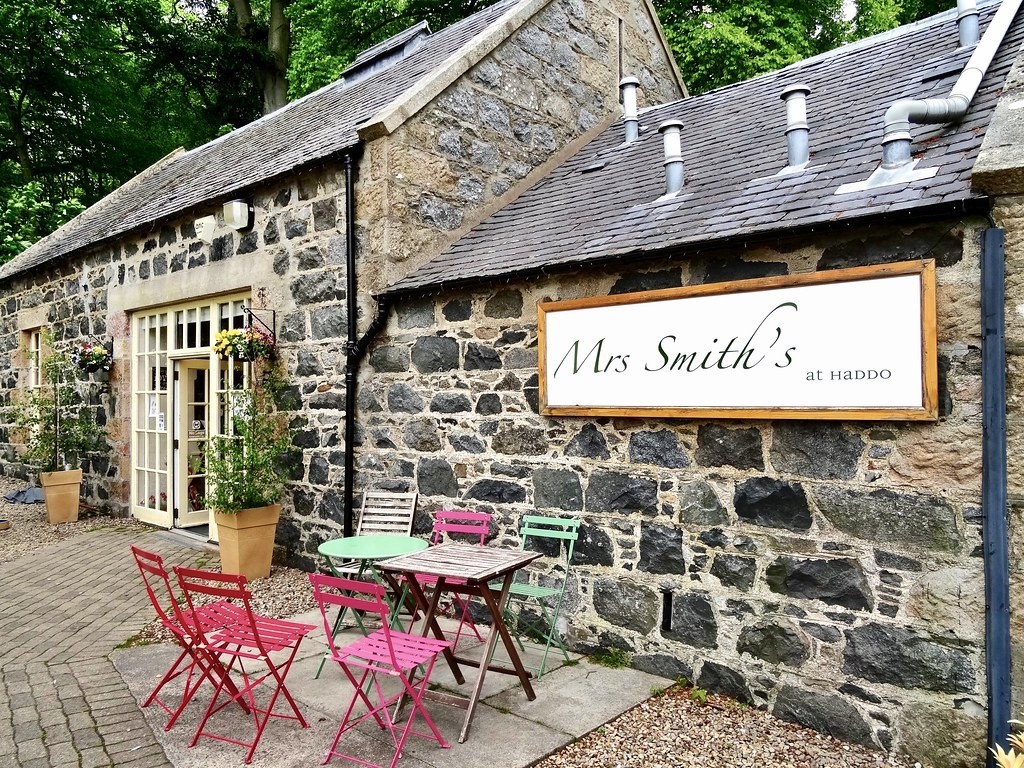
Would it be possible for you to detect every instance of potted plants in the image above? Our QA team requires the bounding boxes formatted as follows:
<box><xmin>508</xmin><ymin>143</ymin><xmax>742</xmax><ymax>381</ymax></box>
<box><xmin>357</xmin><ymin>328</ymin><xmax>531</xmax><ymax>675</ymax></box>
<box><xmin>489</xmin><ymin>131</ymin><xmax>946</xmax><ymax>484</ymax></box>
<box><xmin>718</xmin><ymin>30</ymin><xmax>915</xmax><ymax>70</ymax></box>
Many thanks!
<box><xmin>21</xmin><ymin>327</ymin><xmax>105</xmax><ymax>524</ymax></box>
<box><xmin>190</xmin><ymin>386</ymin><xmax>303</xmax><ymax>579</ymax></box>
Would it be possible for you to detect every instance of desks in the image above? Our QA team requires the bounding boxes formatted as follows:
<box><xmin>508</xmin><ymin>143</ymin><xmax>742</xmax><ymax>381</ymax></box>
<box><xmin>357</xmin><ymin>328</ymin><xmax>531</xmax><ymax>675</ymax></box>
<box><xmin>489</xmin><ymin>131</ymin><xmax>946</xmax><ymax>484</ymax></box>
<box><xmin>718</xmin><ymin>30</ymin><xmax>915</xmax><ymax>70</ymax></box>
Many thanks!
<box><xmin>316</xmin><ymin>534</ymin><xmax>429</xmax><ymax>678</ymax></box>
<box><xmin>374</xmin><ymin>541</ymin><xmax>545</xmax><ymax>742</ymax></box>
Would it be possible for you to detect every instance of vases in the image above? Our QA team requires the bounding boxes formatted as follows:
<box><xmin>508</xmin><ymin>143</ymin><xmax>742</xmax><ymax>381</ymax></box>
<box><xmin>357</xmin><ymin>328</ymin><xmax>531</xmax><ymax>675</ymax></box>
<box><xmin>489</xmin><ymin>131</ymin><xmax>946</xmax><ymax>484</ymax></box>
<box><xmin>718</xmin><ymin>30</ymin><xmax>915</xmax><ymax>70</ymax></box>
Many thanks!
<box><xmin>79</xmin><ymin>360</ymin><xmax>99</xmax><ymax>373</ymax></box>
<box><xmin>216</xmin><ymin>342</ymin><xmax>255</xmax><ymax>362</ymax></box>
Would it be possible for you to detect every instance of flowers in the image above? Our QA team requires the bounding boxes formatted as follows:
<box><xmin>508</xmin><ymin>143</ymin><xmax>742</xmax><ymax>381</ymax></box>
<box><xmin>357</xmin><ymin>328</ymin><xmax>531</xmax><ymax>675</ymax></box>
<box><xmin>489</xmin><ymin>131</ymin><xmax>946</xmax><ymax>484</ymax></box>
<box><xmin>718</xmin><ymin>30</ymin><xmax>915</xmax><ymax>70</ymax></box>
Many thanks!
<box><xmin>212</xmin><ymin>327</ymin><xmax>275</xmax><ymax>360</ymax></box>
<box><xmin>72</xmin><ymin>344</ymin><xmax>113</xmax><ymax>370</ymax></box>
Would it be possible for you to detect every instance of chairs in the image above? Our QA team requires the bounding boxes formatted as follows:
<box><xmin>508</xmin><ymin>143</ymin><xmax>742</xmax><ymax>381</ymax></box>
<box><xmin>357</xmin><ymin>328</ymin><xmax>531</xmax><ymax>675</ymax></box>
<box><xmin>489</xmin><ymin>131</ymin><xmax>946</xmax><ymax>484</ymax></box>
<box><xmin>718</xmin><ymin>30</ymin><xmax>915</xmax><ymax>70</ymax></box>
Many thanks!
<box><xmin>130</xmin><ymin>491</ymin><xmax>581</xmax><ymax>768</ymax></box>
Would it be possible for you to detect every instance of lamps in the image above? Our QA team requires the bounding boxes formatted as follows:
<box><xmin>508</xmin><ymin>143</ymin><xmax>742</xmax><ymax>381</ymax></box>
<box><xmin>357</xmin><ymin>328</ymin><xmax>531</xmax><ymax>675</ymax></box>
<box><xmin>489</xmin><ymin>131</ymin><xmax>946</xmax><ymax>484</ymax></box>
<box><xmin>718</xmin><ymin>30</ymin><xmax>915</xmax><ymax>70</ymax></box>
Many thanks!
<box><xmin>223</xmin><ymin>198</ymin><xmax>255</xmax><ymax>234</ymax></box>
